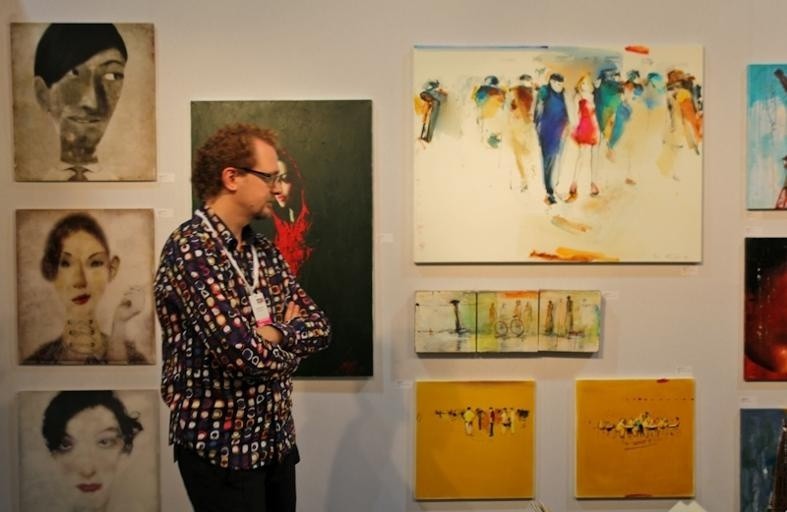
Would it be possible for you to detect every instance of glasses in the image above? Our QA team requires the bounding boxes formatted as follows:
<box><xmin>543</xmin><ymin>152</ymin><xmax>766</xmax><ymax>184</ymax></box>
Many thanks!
<box><xmin>239</xmin><ymin>168</ymin><xmax>281</xmax><ymax>184</ymax></box>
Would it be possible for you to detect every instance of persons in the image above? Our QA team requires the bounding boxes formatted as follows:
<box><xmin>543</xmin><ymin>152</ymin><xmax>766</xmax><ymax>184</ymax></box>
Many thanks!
<box><xmin>20</xmin><ymin>206</ymin><xmax>154</xmax><ymax>362</ymax></box>
<box><xmin>151</xmin><ymin>122</ymin><xmax>330</xmax><ymax>509</ymax></box>
<box><xmin>597</xmin><ymin>402</ymin><xmax>684</xmax><ymax>450</ymax></box>
<box><xmin>472</xmin><ymin>298</ymin><xmax>596</xmax><ymax>347</ymax></box>
<box><xmin>441</xmin><ymin>400</ymin><xmax>533</xmax><ymax>437</ymax></box>
<box><xmin>30</xmin><ymin>386</ymin><xmax>144</xmax><ymax>510</ymax></box>
<box><xmin>24</xmin><ymin>23</ymin><xmax>131</xmax><ymax>184</ymax></box>
<box><xmin>250</xmin><ymin>148</ymin><xmax>329</xmax><ymax>382</ymax></box>
